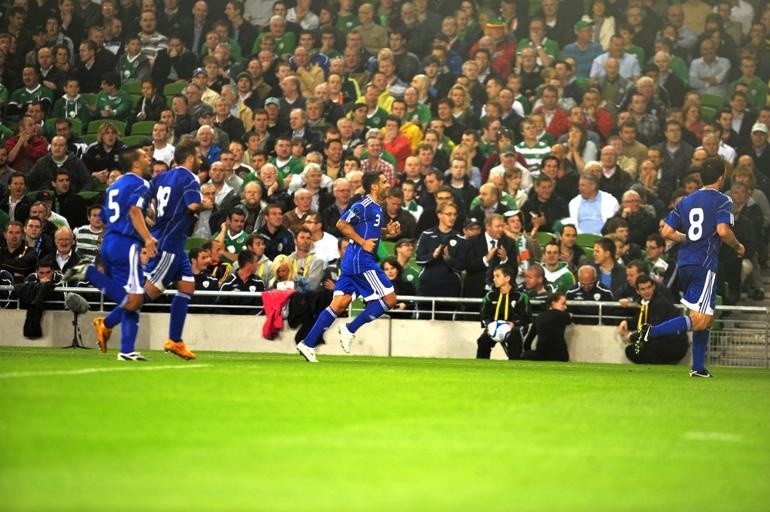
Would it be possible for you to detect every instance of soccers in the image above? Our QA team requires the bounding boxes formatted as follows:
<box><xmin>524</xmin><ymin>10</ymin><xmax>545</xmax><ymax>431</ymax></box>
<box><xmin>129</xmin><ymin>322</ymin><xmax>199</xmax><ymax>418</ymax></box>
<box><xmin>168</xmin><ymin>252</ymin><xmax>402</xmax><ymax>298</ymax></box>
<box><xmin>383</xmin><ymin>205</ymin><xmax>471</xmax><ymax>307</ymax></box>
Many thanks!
<box><xmin>487</xmin><ymin>320</ymin><xmax>511</xmax><ymax>342</ymax></box>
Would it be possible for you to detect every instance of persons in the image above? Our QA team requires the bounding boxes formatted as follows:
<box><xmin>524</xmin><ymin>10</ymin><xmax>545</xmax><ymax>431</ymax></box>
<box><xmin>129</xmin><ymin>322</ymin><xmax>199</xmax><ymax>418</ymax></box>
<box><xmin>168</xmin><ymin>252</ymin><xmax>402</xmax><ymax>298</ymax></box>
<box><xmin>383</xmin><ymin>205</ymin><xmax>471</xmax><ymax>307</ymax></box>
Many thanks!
<box><xmin>1</xmin><ymin>1</ymin><xmax>769</xmax><ymax>377</ymax></box>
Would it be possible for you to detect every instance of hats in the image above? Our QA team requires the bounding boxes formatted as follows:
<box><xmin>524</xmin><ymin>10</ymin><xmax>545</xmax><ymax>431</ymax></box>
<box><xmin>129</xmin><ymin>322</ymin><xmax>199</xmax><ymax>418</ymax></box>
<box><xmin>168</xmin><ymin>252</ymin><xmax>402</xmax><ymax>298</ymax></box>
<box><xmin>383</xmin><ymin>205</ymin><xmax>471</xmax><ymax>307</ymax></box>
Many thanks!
<box><xmin>751</xmin><ymin>121</ymin><xmax>768</xmax><ymax>137</ymax></box>
<box><xmin>573</xmin><ymin>20</ymin><xmax>595</xmax><ymax>34</ymax></box>
<box><xmin>500</xmin><ymin>142</ymin><xmax>517</xmax><ymax>158</ymax></box>
<box><xmin>486</xmin><ymin>17</ymin><xmax>507</xmax><ymax>29</ymax></box>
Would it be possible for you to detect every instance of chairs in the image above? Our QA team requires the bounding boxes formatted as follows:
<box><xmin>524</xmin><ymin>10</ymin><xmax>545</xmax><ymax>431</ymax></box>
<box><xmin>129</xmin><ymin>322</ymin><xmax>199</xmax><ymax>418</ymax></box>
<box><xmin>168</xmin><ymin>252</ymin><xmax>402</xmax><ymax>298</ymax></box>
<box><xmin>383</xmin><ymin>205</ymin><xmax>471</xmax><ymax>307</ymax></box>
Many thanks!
<box><xmin>1</xmin><ymin>78</ymin><xmax>213</xmax><ymax>256</ymax></box>
<box><xmin>574</xmin><ymin>44</ymin><xmax>732</xmax><ymax>125</ymax></box>
<box><xmin>380</xmin><ymin>224</ymin><xmax>605</xmax><ymax>293</ymax></box>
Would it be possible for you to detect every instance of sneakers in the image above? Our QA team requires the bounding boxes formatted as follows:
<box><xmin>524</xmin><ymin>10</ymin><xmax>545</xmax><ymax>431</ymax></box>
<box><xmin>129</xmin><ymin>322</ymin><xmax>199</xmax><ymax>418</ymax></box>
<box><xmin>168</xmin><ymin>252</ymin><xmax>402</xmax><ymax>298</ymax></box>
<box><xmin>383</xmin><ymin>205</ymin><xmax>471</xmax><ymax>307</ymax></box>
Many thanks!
<box><xmin>635</xmin><ymin>323</ymin><xmax>652</xmax><ymax>357</ymax></box>
<box><xmin>93</xmin><ymin>315</ymin><xmax>113</xmax><ymax>354</ymax></box>
<box><xmin>690</xmin><ymin>366</ymin><xmax>713</xmax><ymax>378</ymax></box>
<box><xmin>165</xmin><ymin>338</ymin><xmax>196</xmax><ymax>362</ymax></box>
<box><xmin>116</xmin><ymin>350</ymin><xmax>153</xmax><ymax>362</ymax></box>
<box><xmin>296</xmin><ymin>340</ymin><xmax>319</xmax><ymax>364</ymax></box>
<box><xmin>339</xmin><ymin>322</ymin><xmax>356</xmax><ymax>354</ymax></box>
